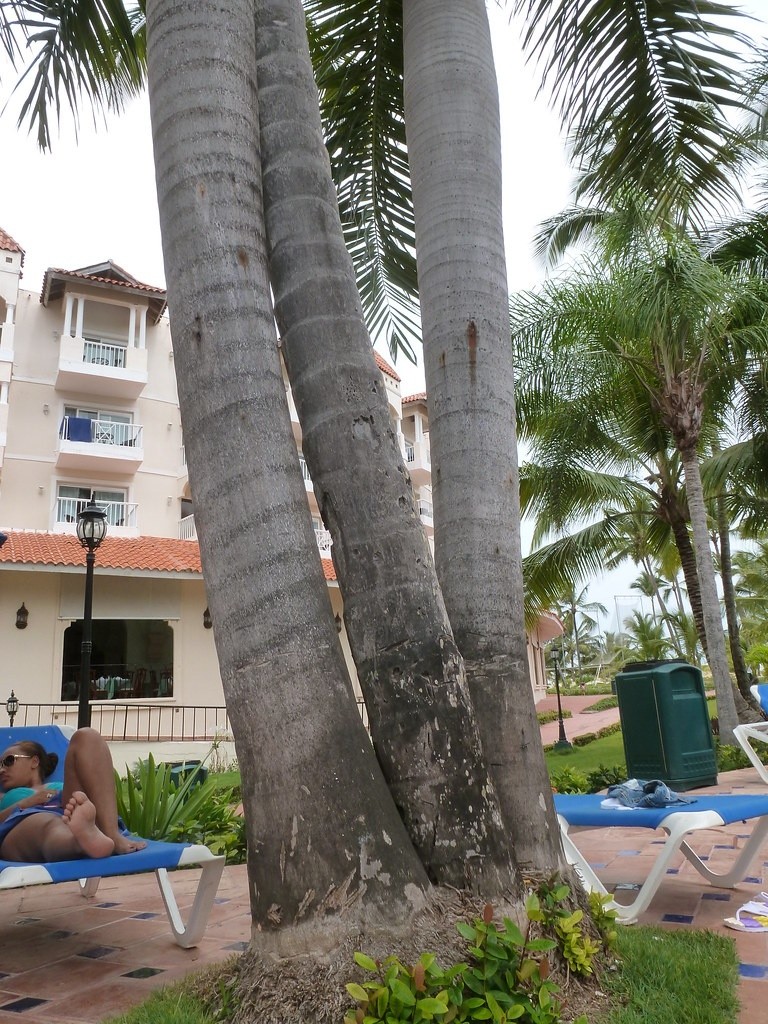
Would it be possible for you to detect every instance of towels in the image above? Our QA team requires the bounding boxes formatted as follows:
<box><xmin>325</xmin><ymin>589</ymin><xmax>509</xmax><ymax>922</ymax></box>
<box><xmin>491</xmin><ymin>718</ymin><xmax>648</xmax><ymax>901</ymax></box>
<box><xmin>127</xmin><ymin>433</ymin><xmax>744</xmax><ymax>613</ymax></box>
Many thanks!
<box><xmin>58</xmin><ymin>415</ymin><xmax>92</xmax><ymax>443</ymax></box>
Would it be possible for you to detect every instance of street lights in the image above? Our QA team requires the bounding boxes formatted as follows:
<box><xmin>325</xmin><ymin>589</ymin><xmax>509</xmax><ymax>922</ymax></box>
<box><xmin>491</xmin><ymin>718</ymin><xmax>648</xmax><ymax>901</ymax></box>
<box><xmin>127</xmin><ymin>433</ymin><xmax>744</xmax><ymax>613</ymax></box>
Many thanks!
<box><xmin>76</xmin><ymin>493</ymin><xmax>109</xmax><ymax>730</ymax></box>
<box><xmin>550</xmin><ymin>644</ymin><xmax>571</xmax><ymax>747</ymax></box>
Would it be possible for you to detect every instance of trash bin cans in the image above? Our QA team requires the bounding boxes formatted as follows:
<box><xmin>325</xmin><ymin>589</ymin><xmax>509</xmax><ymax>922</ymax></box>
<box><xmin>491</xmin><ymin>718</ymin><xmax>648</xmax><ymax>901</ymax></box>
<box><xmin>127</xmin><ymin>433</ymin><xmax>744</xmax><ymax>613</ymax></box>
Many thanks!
<box><xmin>613</xmin><ymin>657</ymin><xmax>719</xmax><ymax>793</ymax></box>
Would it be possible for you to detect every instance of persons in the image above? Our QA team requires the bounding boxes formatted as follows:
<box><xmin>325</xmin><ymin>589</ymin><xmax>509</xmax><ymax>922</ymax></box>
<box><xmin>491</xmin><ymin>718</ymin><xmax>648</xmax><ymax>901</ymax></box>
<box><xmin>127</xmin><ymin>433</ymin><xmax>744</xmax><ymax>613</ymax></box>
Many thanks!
<box><xmin>0</xmin><ymin>728</ymin><xmax>147</xmax><ymax>862</ymax></box>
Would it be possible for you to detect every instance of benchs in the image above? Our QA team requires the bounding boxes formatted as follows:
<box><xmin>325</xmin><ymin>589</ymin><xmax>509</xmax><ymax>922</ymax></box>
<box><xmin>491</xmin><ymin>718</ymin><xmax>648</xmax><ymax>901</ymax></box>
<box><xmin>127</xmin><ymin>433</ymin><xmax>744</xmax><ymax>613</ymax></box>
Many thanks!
<box><xmin>553</xmin><ymin>790</ymin><xmax>768</xmax><ymax>925</ymax></box>
<box><xmin>0</xmin><ymin>725</ymin><xmax>227</xmax><ymax>951</ymax></box>
<box><xmin>732</xmin><ymin>684</ymin><xmax>768</xmax><ymax>784</ymax></box>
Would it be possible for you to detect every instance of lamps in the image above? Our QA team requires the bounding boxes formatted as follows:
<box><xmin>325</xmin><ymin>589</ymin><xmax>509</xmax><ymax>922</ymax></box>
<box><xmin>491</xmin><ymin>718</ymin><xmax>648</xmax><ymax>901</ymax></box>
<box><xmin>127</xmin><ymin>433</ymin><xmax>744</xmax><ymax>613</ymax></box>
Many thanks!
<box><xmin>203</xmin><ymin>606</ymin><xmax>212</xmax><ymax>629</ymax></box>
<box><xmin>335</xmin><ymin>612</ymin><xmax>341</xmax><ymax>633</ymax></box>
<box><xmin>16</xmin><ymin>602</ymin><xmax>30</xmax><ymax>629</ymax></box>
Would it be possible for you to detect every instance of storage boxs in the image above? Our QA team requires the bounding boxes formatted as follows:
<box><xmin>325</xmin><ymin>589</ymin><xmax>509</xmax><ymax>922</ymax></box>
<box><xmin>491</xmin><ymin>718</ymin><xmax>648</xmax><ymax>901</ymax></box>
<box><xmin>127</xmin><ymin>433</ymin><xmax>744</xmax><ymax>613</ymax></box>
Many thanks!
<box><xmin>615</xmin><ymin>657</ymin><xmax>720</xmax><ymax>792</ymax></box>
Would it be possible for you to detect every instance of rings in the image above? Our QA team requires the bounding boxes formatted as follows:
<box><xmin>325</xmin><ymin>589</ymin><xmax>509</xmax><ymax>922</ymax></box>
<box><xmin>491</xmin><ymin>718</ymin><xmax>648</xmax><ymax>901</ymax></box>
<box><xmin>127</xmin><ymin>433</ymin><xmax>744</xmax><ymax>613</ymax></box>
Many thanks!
<box><xmin>47</xmin><ymin>793</ymin><xmax>53</xmax><ymax>798</ymax></box>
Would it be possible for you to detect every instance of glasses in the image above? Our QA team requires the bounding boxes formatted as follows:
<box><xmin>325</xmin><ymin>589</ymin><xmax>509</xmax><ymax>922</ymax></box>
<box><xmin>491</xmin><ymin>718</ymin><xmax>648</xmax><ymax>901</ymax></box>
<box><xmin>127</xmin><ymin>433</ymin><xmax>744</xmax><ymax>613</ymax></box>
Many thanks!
<box><xmin>0</xmin><ymin>754</ymin><xmax>32</xmax><ymax>769</ymax></box>
